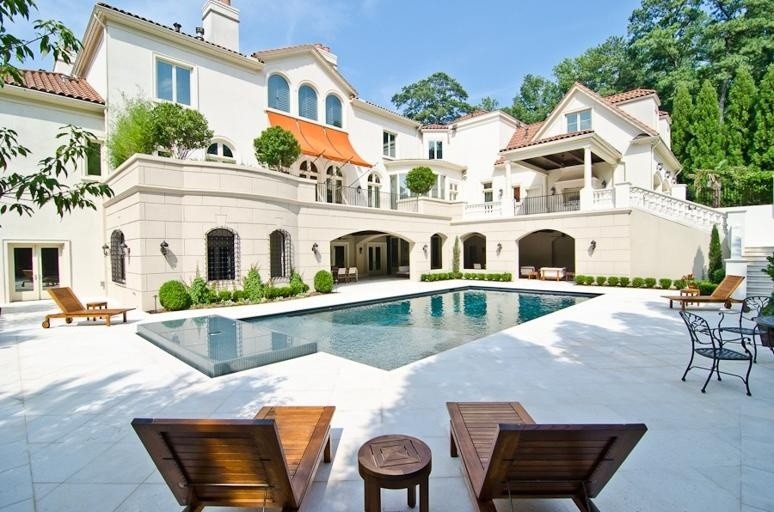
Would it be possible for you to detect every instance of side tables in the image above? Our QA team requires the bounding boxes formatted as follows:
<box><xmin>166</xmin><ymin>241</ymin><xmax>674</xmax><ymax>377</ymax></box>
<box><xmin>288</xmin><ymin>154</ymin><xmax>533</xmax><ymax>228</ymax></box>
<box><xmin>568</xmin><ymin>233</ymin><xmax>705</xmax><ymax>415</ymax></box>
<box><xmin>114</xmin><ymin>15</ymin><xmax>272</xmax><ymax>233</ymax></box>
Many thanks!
<box><xmin>87</xmin><ymin>302</ymin><xmax>107</xmax><ymax>321</ymax></box>
<box><xmin>528</xmin><ymin>272</ymin><xmax>539</xmax><ymax>280</ymax></box>
<box><xmin>357</xmin><ymin>434</ymin><xmax>432</xmax><ymax>512</ymax></box>
<box><xmin>680</xmin><ymin>289</ymin><xmax>700</xmax><ymax>307</ymax></box>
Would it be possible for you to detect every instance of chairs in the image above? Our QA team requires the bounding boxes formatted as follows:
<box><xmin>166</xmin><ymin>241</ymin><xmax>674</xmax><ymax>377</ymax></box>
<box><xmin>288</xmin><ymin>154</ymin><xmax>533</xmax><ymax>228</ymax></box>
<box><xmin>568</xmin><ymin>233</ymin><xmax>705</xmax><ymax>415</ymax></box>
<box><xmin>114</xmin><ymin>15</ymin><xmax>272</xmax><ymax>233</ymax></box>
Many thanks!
<box><xmin>679</xmin><ymin>297</ymin><xmax>773</xmax><ymax>396</ymax></box>
<box><xmin>662</xmin><ymin>275</ymin><xmax>745</xmax><ymax>312</ymax></box>
<box><xmin>42</xmin><ymin>288</ymin><xmax>136</xmax><ymax>327</ymax></box>
<box><xmin>131</xmin><ymin>407</ymin><xmax>333</xmax><ymax>510</ymax></box>
<box><xmin>446</xmin><ymin>401</ymin><xmax>648</xmax><ymax>512</ymax></box>
<box><xmin>337</xmin><ymin>267</ymin><xmax>358</xmax><ymax>283</ymax></box>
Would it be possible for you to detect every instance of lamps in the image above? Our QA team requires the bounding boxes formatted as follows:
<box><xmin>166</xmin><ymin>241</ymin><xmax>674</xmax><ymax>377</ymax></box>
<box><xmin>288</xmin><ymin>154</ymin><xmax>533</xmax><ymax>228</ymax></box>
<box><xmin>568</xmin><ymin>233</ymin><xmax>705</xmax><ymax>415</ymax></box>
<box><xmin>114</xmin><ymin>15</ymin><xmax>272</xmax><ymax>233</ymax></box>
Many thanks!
<box><xmin>160</xmin><ymin>240</ymin><xmax>168</xmax><ymax>255</ymax></box>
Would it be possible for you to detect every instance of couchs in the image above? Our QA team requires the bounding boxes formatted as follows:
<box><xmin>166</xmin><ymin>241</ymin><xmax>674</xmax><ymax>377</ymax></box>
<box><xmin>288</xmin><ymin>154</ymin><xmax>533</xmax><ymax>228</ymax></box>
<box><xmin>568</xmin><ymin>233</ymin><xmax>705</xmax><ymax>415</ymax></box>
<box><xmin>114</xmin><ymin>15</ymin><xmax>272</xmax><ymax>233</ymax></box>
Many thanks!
<box><xmin>540</xmin><ymin>266</ymin><xmax>566</xmax><ymax>282</ymax></box>
